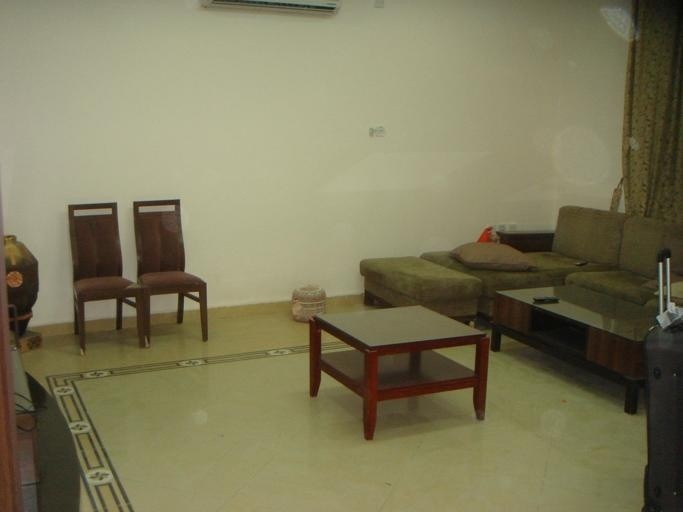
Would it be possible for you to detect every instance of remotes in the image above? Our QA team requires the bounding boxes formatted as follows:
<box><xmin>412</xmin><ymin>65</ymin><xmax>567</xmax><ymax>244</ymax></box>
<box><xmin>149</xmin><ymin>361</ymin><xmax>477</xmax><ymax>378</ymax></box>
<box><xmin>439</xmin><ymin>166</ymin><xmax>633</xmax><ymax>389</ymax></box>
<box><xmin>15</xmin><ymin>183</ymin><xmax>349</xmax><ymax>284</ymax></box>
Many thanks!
<box><xmin>533</xmin><ymin>297</ymin><xmax>560</xmax><ymax>301</ymax></box>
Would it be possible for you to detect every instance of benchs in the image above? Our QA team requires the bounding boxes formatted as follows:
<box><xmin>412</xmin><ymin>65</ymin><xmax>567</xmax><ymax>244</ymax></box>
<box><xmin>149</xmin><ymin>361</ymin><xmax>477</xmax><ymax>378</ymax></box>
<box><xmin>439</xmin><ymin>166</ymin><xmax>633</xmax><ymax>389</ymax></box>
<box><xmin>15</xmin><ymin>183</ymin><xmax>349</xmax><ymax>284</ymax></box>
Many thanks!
<box><xmin>359</xmin><ymin>257</ymin><xmax>482</xmax><ymax>327</ymax></box>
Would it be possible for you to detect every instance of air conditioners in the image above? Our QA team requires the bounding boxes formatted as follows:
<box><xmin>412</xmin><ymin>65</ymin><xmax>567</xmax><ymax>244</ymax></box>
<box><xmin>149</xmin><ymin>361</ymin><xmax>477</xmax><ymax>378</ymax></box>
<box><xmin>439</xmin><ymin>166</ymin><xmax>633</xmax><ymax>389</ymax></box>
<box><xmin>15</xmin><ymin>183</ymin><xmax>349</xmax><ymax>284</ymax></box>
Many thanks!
<box><xmin>202</xmin><ymin>1</ymin><xmax>340</xmax><ymax>17</ymax></box>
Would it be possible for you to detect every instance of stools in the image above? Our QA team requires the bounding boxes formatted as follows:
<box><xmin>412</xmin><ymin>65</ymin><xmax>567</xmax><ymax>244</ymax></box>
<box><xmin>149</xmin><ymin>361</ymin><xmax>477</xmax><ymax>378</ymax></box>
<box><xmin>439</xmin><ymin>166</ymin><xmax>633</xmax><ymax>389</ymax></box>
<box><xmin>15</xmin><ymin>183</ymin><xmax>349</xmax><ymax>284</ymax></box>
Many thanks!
<box><xmin>290</xmin><ymin>284</ymin><xmax>326</xmax><ymax>323</ymax></box>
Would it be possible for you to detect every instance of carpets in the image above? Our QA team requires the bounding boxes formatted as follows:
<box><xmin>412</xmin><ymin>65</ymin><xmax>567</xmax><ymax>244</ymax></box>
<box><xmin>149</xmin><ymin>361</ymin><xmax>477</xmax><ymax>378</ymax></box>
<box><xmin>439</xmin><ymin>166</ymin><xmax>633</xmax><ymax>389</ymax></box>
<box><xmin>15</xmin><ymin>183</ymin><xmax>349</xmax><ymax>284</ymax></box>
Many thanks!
<box><xmin>47</xmin><ymin>342</ymin><xmax>654</xmax><ymax>512</ymax></box>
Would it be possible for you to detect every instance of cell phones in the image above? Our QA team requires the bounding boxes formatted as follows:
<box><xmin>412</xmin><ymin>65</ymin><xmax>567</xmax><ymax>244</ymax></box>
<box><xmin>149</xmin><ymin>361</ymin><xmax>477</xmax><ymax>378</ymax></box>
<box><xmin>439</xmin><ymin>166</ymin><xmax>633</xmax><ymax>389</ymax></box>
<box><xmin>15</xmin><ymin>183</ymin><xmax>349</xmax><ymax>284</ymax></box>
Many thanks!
<box><xmin>575</xmin><ymin>261</ymin><xmax>587</xmax><ymax>265</ymax></box>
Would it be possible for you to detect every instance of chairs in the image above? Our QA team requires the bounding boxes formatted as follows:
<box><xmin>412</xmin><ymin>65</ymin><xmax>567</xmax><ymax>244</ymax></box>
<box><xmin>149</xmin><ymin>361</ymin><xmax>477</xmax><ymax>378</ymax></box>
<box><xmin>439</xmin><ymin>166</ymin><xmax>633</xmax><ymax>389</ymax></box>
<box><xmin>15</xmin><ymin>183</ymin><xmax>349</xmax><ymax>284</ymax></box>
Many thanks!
<box><xmin>68</xmin><ymin>201</ymin><xmax>209</xmax><ymax>354</ymax></box>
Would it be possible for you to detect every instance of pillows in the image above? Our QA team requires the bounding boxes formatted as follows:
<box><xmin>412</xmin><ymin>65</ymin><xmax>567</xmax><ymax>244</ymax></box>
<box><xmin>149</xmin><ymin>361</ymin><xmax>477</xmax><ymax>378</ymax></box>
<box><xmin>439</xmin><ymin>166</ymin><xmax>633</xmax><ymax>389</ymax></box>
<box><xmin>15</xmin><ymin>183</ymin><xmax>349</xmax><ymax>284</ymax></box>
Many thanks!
<box><xmin>449</xmin><ymin>243</ymin><xmax>538</xmax><ymax>272</ymax></box>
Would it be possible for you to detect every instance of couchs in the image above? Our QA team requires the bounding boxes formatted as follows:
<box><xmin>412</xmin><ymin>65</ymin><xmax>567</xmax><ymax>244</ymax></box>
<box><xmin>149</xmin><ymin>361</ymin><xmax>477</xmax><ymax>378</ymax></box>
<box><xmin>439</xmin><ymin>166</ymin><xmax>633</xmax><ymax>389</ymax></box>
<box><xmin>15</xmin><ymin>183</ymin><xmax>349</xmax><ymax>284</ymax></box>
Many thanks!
<box><xmin>421</xmin><ymin>205</ymin><xmax>682</xmax><ymax>322</ymax></box>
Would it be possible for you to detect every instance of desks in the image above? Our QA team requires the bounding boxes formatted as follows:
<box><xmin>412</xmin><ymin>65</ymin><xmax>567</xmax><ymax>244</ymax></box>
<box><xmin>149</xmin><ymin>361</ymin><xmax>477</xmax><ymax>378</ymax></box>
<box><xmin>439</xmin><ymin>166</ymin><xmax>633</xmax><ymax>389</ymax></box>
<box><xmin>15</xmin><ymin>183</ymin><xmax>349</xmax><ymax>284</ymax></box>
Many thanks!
<box><xmin>308</xmin><ymin>305</ymin><xmax>491</xmax><ymax>441</ymax></box>
